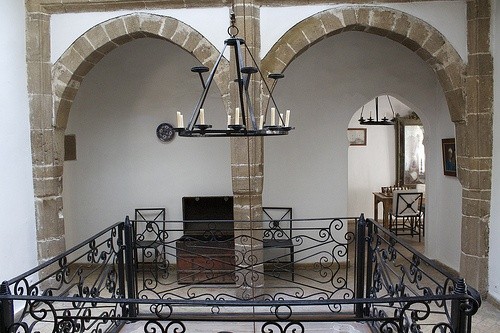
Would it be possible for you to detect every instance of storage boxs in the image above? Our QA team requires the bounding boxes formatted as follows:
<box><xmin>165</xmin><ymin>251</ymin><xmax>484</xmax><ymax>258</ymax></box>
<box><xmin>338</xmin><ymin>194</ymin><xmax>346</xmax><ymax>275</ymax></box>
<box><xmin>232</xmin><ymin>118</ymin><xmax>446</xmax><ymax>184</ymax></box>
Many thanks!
<box><xmin>175</xmin><ymin>195</ymin><xmax>236</xmax><ymax>287</ymax></box>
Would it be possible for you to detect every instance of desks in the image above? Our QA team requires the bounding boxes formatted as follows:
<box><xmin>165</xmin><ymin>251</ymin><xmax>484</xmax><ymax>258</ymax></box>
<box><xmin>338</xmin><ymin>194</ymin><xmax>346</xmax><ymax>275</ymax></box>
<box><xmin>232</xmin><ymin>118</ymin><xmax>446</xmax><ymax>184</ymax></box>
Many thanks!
<box><xmin>372</xmin><ymin>192</ymin><xmax>425</xmax><ymax>230</ymax></box>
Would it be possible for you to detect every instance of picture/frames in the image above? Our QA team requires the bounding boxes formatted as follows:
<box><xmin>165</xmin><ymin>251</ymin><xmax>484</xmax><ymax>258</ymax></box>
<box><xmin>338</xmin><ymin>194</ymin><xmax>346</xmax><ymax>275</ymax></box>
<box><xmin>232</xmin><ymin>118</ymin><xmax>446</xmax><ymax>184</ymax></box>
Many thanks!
<box><xmin>347</xmin><ymin>128</ymin><xmax>367</xmax><ymax>145</ymax></box>
<box><xmin>442</xmin><ymin>138</ymin><xmax>457</xmax><ymax>177</ymax></box>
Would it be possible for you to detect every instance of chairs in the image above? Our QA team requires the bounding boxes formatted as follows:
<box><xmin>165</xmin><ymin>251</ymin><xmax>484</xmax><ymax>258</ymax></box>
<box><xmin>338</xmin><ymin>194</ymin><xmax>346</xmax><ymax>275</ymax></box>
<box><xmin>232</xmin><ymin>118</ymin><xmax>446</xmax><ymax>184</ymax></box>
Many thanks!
<box><xmin>389</xmin><ymin>193</ymin><xmax>424</xmax><ymax>243</ymax></box>
<box><xmin>134</xmin><ymin>208</ymin><xmax>167</xmax><ymax>270</ymax></box>
<box><xmin>262</xmin><ymin>207</ymin><xmax>296</xmax><ymax>282</ymax></box>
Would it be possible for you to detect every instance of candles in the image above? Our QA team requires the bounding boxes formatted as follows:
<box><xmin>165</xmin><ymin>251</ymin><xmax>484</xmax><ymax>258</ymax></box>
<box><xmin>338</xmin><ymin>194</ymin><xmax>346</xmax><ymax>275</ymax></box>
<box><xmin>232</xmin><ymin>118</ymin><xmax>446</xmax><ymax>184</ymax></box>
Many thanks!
<box><xmin>235</xmin><ymin>108</ymin><xmax>239</xmax><ymax>125</ymax></box>
<box><xmin>176</xmin><ymin>111</ymin><xmax>184</xmax><ymax>128</ymax></box>
<box><xmin>370</xmin><ymin>111</ymin><xmax>372</xmax><ymax>118</ymax></box>
<box><xmin>279</xmin><ymin>114</ymin><xmax>283</xmax><ymax>127</ymax></box>
<box><xmin>259</xmin><ymin>115</ymin><xmax>264</xmax><ymax>129</ymax></box>
<box><xmin>227</xmin><ymin>115</ymin><xmax>231</xmax><ymax>130</ymax></box>
<box><xmin>384</xmin><ymin>113</ymin><xmax>386</xmax><ymax>117</ymax></box>
<box><xmin>285</xmin><ymin>110</ymin><xmax>291</xmax><ymax>126</ymax></box>
<box><xmin>200</xmin><ymin>109</ymin><xmax>204</xmax><ymax>124</ymax></box>
<box><xmin>270</xmin><ymin>108</ymin><xmax>275</xmax><ymax>125</ymax></box>
